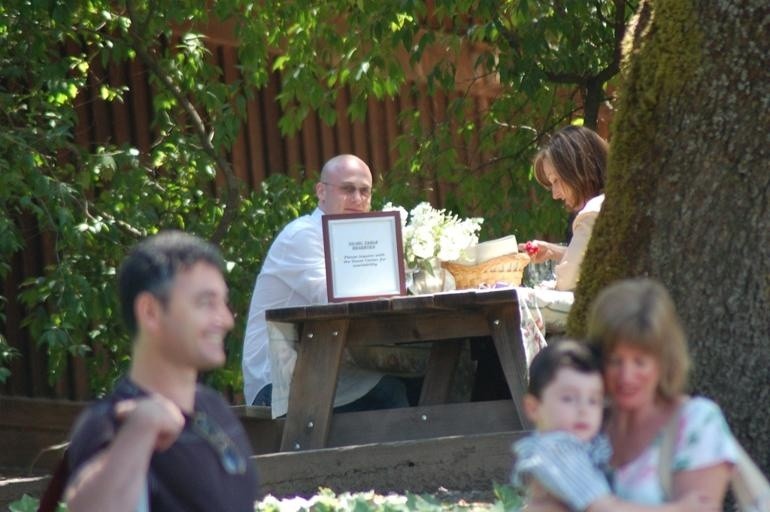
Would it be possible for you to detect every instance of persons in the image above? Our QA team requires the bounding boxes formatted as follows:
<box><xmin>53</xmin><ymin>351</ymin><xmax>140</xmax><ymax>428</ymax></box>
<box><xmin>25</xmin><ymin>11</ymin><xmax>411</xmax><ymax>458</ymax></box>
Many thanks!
<box><xmin>587</xmin><ymin>279</ymin><xmax>740</xmax><ymax>512</ymax></box>
<box><xmin>509</xmin><ymin>341</ymin><xmax>629</xmax><ymax>512</ymax></box>
<box><xmin>241</xmin><ymin>154</ymin><xmax>408</xmax><ymax>414</ymax></box>
<box><xmin>36</xmin><ymin>233</ymin><xmax>260</xmax><ymax>511</ymax></box>
<box><xmin>517</xmin><ymin>125</ymin><xmax>609</xmax><ymax>290</ymax></box>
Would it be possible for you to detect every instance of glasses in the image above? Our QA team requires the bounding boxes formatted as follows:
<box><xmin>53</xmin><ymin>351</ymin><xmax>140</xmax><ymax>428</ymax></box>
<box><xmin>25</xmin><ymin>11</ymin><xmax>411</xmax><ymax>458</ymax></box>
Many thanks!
<box><xmin>322</xmin><ymin>182</ymin><xmax>374</xmax><ymax>197</ymax></box>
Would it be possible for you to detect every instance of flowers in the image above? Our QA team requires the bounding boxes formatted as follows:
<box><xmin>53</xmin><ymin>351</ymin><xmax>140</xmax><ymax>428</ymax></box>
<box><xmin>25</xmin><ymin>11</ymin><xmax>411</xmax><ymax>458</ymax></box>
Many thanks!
<box><xmin>381</xmin><ymin>202</ymin><xmax>484</xmax><ymax>278</ymax></box>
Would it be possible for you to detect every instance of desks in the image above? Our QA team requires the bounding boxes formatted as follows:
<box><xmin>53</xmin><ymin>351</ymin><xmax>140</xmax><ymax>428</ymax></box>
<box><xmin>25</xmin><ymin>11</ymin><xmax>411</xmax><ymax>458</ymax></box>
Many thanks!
<box><xmin>265</xmin><ymin>288</ymin><xmax>574</xmax><ymax>453</ymax></box>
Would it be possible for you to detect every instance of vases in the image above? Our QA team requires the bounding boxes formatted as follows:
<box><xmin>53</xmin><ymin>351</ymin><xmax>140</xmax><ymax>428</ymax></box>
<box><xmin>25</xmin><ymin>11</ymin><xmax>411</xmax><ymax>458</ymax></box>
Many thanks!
<box><xmin>408</xmin><ymin>258</ymin><xmax>444</xmax><ymax>294</ymax></box>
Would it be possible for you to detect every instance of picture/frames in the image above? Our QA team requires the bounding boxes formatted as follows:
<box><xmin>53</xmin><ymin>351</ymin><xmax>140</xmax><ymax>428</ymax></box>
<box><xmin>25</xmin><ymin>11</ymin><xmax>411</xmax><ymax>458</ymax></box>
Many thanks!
<box><xmin>320</xmin><ymin>212</ymin><xmax>405</xmax><ymax>304</ymax></box>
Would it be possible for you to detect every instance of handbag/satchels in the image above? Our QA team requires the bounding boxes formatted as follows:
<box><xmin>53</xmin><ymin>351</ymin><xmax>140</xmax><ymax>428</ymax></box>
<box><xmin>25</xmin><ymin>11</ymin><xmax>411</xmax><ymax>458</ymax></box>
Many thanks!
<box><xmin>660</xmin><ymin>399</ymin><xmax>770</xmax><ymax>512</ymax></box>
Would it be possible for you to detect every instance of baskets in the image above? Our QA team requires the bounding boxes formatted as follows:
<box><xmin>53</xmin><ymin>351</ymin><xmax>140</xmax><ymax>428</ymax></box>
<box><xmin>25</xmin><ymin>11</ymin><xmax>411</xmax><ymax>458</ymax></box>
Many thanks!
<box><xmin>440</xmin><ymin>252</ymin><xmax>531</xmax><ymax>290</ymax></box>
